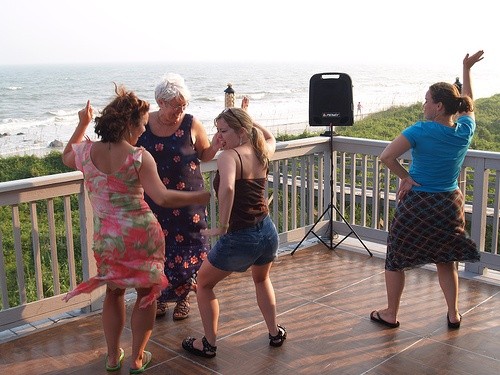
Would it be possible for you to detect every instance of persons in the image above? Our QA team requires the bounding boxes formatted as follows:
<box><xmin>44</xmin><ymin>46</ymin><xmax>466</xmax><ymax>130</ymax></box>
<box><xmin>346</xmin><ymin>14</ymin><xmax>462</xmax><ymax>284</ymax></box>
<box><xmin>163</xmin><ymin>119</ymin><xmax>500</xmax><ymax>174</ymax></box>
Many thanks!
<box><xmin>60</xmin><ymin>80</ymin><xmax>211</xmax><ymax>374</ymax></box>
<box><xmin>369</xmin><ymin>49</ymin><xmax>485</xmax><ymax>329</ymax></box>
<box><xmin>181</xmin><ymin>96</ymin><xmax>286</xmax><ymax>360</ymax></box>
<box><xmin>131</xmin><ymin>81</ymin><xmax>222</xmax><ymax>321</ymax></box>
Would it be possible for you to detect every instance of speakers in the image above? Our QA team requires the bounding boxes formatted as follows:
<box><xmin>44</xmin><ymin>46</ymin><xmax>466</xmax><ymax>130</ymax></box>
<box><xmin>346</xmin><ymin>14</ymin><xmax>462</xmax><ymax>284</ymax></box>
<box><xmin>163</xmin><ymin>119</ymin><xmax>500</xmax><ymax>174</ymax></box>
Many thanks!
<box><xmin>309</xmin><ymin>72</ymin><xmax>354</xmax><ymax>127</ymax></box>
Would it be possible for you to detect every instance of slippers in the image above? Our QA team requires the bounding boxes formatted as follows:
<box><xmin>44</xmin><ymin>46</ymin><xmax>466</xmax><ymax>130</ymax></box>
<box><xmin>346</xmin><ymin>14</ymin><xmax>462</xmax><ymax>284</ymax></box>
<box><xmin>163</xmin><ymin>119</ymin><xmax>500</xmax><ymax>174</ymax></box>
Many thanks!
<box><xmin>129</xmin><ymin>351</ymin><xmax>152</xmax><ymax>373</ymax></box>
<box><xmin>447</xmin><ymin>310</ymin><xmax>462</xmax><ymax>329</ymax></box>
<box><xmin>370</xmin><ymin>310</ymin><xmax>400</xmax><ymax>328</ymax></box>
<box><xmin>105</xmin><ymin>347</ymin><xmax>124</xmax><ymax>372</ymax></box>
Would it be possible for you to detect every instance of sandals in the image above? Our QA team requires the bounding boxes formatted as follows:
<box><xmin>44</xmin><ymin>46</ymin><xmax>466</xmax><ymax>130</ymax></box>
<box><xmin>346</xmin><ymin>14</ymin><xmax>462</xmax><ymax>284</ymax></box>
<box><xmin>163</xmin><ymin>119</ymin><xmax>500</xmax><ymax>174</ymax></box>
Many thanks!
<box><xmin>155</xmin><ymin>300</ymin><xmax>169</xmax><ymax>317</ymax></box>
<box><xmin>181</xmin><ymin>336</ymin><xmax>217</xmax><ymax>358</ymax></box>
<box><xmin>268</xmin><ymin>324</ymin><xmax>287</xmax><ymax>347</ymax></box>
<box><xmin>172</xmin><ymin>296</ymin><xmax>191</xmax><ymax>319</ymax></box>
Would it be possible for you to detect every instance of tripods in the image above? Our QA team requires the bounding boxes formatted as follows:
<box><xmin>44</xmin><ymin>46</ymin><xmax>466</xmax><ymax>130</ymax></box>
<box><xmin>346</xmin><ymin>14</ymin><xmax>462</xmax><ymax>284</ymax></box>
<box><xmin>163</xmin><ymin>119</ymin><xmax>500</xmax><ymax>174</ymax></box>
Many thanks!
<box><xmin>291</xmin><ymin>127</ymin><xmax>373</xmax><ymax>256</ymax></box>
